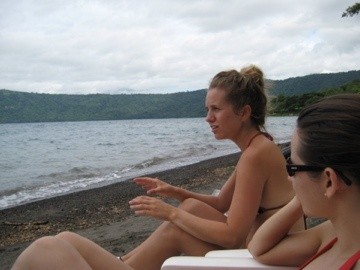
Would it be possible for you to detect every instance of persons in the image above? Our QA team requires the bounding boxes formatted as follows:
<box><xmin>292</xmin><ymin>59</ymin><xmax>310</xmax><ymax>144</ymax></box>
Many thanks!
<box><xmin>116</xmin><ymin>64</ymin><xmax>308</xmax><ymax>269</ymax></box>
<box><xmin>12</xmin><ymin>92</ymin><xmax>360</xmax><ymax>270</ymax></box>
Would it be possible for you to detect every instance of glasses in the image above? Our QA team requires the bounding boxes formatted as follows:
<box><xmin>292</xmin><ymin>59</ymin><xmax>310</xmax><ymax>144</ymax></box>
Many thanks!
<box><xmin>285</xmin><ymin>157</ymin><xmax>355</xmax><ymax>187</ymax></box>
